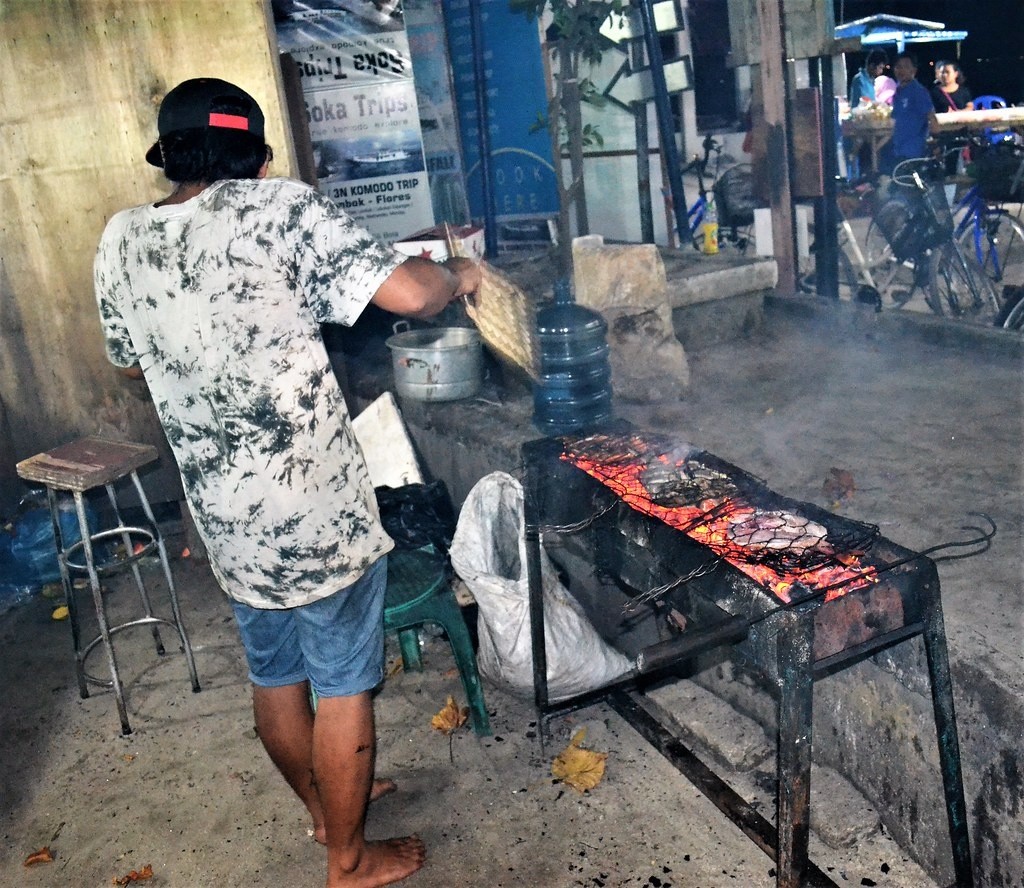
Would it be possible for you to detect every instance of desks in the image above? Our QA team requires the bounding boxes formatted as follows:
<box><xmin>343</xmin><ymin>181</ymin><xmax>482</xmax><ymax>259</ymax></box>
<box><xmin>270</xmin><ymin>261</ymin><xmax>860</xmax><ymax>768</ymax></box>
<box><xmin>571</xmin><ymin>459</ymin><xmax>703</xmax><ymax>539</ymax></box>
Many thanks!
<box><xmin>841</xmin><ymin>107</ymin><xmax>1023</xmax><ymax>186</ymax></box>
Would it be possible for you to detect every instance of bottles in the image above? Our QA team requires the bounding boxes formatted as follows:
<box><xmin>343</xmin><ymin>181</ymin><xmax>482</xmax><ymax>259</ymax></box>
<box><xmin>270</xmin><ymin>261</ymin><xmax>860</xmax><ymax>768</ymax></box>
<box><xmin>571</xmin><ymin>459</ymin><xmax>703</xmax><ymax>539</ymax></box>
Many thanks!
<box><xmin>532</xmin><ymin>274</ymin><xmax>616</xmax><ymax>438</ymax></box>
<box><xmin>702</xmin><ymin>204</ymin><xmax>719</xmax><ymax>255</ymax></box>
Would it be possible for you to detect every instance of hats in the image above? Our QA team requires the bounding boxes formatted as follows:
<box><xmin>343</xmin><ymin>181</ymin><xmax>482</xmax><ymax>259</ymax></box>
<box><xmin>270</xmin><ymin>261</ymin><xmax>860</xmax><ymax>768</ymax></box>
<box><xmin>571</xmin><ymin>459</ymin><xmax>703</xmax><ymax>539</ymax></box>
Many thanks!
<box><xmin>147</xmin><ymin>78</ymin><xmax>265</xmax><ymax>167</ymax></box>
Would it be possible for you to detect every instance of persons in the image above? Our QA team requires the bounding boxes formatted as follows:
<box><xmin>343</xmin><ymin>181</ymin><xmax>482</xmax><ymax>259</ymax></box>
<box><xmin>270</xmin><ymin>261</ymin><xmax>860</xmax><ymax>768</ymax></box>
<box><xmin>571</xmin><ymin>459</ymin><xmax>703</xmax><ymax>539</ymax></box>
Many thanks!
<box><xmin>926</xmin><ymin>57</ymin><xmax>975</xmax><ymax>178</ymax></box>
<box><xmin>92</xmin><ymin>76</ymin><xmax>482</xmax><ymax>888</ymax></box>
<box><xmin>881</xmin><ymin>50</ymin><xmax>940</xmax><ymax>192</ymax></box>
<box><xmin>846</xmin><ymin>48</ymin><xmax>888</xmax><ymax>182</ymax></box>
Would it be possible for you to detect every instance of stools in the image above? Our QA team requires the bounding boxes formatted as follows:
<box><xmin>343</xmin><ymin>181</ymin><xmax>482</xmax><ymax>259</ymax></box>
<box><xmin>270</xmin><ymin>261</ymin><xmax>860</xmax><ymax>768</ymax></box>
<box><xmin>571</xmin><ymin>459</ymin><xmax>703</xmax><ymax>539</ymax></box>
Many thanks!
<box><xmin>370</xmin><ymin>548</ymin><xmax>496</xmax><ymax>739</ymax></box>
<box><xmin>15</xmin><ymin>436</ymin><xmax>206</xmax><ymax>738</ymax></box>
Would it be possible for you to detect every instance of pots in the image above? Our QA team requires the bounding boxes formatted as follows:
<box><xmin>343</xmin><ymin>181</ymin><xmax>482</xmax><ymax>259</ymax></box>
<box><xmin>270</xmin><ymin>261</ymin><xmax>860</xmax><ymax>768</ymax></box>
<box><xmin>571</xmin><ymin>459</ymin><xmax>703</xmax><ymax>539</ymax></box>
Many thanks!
<box><xmin>383</xmin><ymin>318</ymin><xmax>488</xmax><ymax>403</ymax></box>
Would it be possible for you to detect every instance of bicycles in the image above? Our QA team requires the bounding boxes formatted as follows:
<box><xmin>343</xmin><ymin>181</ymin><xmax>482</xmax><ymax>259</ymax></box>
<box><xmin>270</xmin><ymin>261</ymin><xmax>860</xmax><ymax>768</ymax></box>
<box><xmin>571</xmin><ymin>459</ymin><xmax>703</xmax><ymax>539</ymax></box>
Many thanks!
<box><xmin>674</xmin><ymin>133</ymin><xmax>758</xmax><ymax>258</ymax></box>
<box><xmin>791</xmin><ymin>121</ymin><xmax>1024</xmax><ymax>334</ymax></box>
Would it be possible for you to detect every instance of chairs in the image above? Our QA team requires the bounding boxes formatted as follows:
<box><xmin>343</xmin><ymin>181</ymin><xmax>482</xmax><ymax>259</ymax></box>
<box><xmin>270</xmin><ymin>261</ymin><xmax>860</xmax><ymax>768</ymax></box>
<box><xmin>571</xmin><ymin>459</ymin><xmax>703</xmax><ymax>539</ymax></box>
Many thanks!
<box><xmin>969</xmin><ymin>97</ymin><xmax>1013</xmax><ymax>146</ymax></box>
